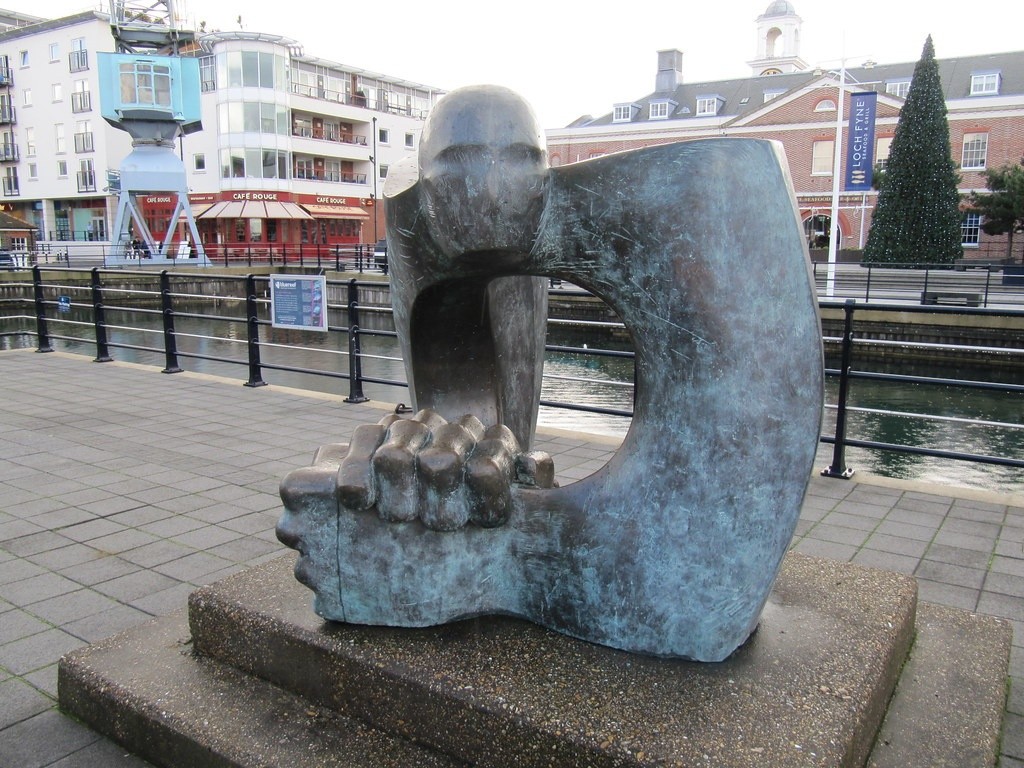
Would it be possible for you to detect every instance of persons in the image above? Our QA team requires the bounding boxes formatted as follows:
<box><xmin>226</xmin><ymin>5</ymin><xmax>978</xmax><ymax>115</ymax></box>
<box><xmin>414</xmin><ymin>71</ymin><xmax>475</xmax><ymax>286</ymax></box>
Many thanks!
<box><xmin>124</xmin><ymin>235</ymin><xmax>151</xmax><ymax>259</ymax></box>
<box><xmin>87</xmin><ymin>221</ymin><xmax>94</xmax><ymax>241</ymax></box>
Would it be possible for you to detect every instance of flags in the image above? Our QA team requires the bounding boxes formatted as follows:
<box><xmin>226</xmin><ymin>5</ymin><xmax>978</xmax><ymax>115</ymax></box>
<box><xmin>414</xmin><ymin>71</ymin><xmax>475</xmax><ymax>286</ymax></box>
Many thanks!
<box><xmin>844</xmin><ymin>91</ymin><xmax>876</xmax><ymax>191</ymax></box>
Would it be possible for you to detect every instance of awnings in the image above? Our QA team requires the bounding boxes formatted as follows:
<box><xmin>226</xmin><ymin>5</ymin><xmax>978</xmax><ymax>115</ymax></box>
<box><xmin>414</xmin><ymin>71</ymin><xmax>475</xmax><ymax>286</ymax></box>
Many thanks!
<box><xmin>300</xmin><ymin>204</ymin><xmax>370</xmax><ymax>221</ymax></box>
<box><xmin>167</xmin><ymin>202</ymin><xmax>315</xmax><ymax>225</ymax></box>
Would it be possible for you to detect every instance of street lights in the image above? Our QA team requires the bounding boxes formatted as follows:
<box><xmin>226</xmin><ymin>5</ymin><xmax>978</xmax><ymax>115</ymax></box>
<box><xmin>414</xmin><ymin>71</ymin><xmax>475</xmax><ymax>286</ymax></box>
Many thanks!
<box><xmin>812</xmin><ymin>53</ymin><xmax>877</xmax><ymax>301</ymax></box>
